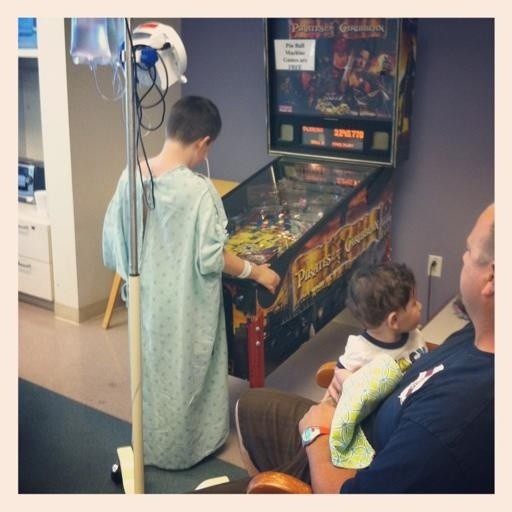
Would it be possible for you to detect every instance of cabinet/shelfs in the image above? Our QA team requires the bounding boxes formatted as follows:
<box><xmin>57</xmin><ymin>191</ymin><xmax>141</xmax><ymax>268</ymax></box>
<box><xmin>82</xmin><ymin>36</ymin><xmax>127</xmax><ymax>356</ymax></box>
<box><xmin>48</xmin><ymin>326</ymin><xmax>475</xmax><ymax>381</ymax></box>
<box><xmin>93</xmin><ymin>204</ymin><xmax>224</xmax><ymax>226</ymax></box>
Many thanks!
<box><xmin>18</xmin><ymin>221</ymin><xmax>53</xmax><ymax>302</ymax></box>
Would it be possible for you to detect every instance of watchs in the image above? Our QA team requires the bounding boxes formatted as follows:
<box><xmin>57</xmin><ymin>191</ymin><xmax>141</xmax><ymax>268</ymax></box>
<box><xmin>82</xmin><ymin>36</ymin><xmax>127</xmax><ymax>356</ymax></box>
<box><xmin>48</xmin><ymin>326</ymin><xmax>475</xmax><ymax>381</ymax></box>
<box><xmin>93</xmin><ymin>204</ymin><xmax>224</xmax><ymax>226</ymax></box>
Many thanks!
<box><xmin>299</xmin><ymin>424</ymin><xmax>329</xmax><ymax>449</ymax></box>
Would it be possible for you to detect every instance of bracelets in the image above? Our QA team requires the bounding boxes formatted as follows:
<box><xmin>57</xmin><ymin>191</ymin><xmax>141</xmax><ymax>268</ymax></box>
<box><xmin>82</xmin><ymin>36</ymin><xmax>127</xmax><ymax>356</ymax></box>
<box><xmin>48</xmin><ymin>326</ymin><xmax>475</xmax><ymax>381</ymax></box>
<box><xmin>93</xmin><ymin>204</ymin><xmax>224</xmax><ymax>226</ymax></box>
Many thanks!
<box><xmin>237</xmin><ymin>260</ymin><xmax>252</xmax><ymax>280</ymax></box>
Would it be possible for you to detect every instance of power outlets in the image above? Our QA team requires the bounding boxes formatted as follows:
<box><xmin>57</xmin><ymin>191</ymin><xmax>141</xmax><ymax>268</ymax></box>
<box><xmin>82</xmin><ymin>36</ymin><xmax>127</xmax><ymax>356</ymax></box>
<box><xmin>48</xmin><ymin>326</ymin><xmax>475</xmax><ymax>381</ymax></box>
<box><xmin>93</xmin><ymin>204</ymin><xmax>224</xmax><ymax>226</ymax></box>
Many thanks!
<box><xmin>428</xmin><ymin>254</ymin><xmax>443</xmax><ymax>278</ymax></box>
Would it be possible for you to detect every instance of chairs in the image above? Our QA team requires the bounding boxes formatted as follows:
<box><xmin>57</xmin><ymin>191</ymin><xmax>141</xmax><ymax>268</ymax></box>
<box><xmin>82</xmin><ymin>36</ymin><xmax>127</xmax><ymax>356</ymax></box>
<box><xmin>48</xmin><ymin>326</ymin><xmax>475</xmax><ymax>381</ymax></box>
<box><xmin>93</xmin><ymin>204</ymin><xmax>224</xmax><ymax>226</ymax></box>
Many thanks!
<box><xmin>246</xmin><ymin>361</ymin><xmax>338</xmax><ymax>493</ymax></box>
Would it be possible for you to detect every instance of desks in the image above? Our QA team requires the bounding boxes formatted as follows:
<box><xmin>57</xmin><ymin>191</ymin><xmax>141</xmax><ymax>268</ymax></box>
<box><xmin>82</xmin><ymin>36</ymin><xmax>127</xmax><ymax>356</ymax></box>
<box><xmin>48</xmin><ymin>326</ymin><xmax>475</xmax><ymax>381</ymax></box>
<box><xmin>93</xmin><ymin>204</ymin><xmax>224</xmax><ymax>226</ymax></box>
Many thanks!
<box><xmin>102</xmin><ymin>180</ymin><xmax>240</xmax><ymax>330</ymax></box>
<box><xmin>420</xmin><ymin>292</ymin><xmax>470</xmax><ymax>351</ymax></box>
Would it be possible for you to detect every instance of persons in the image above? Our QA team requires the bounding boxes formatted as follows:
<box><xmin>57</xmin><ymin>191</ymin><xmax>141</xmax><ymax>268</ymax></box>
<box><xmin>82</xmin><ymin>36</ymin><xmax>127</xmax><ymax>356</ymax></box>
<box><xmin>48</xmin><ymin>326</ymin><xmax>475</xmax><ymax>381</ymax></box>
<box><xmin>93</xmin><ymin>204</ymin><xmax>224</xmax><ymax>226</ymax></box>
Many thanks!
<box><xmin>275</xmin><ymin>34</ymin><xmax>397</xmax><ymax>119</ymax></box>
<box><xmin>101</xmin><ymin>95</ymin><xmax>282</xmax><ymax>471</ymax></box>
<box><xmin>18</xmin><ymin>173</ymin><xmax>33</xmax><ymax>190</ymax></box>
<box><xmin>235</xmin><ymin>204</ymin><xmax>494</xmax><ymax>493</ymax></box>
<box><xmin>328</xmin><ymin>260</ymin><xmax>430</xmax><ymax>403</ymax></box>
